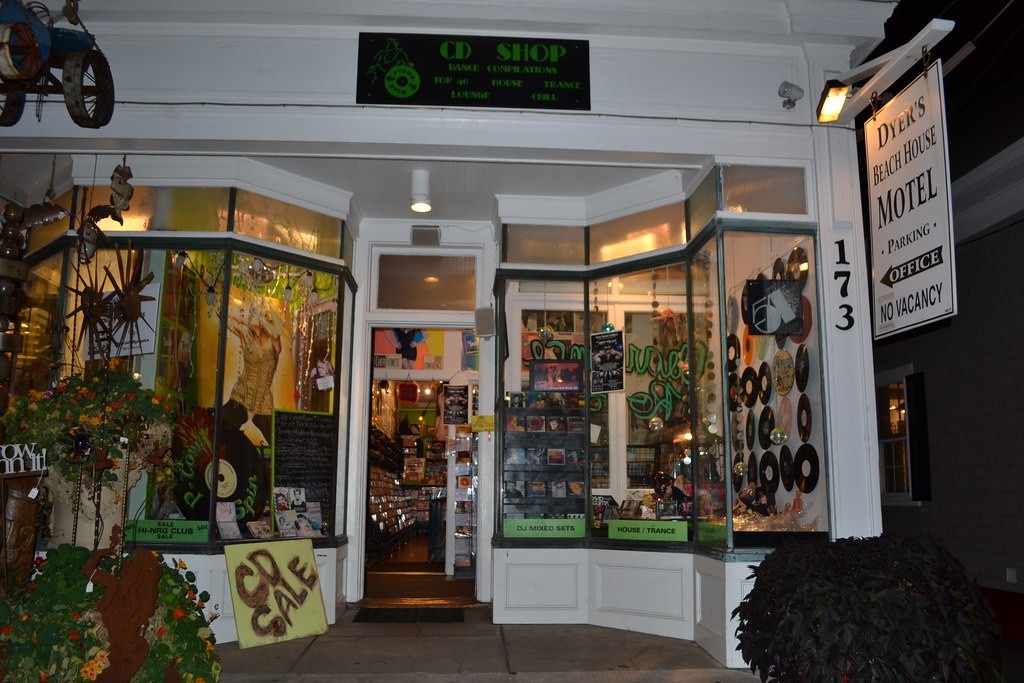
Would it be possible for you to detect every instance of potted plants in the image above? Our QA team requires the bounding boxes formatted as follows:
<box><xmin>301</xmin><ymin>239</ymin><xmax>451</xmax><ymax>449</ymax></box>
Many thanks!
<box><xmin>731</xmin><ymin>531</ymin><xmax>1008</xmax><ymax>683</ymax></box>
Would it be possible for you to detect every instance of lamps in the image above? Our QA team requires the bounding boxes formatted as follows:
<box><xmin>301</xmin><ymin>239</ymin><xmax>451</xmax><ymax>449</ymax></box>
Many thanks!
<box><xmin>779</xmin><ymin>80</ymin><xmax>805</xmax><ymax>110</ymax></box>
<box><xmin>539</xmin><ymin>279</ymin><xmax>555</xmax><ymax>341</ymax></box>
<box><xmin>816</xmin><ymin>79</ymin><xmax>849</xmax><ymax>123</ymax></box>
<box><xmin>409</xmin><ymin>169</ymin><xmax>433</xmax><ymax>213</ymax></box>
<box><xmin>601</xmin><ymin>277</ymin><xmax>617</xmax><ymax>334</ymax></box>
<box><xmin>176</xmin><ymin>248</ymin><xmax>318</xmax><ymax>304</ymax></box>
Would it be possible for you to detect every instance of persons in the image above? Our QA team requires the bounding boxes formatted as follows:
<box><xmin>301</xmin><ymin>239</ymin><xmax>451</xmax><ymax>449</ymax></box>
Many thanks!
<box><xmin>277</xmin><ymin>493</ymin><xmax>291</xmax><ymax>510</ymax></box>
<box><xmin>291</xmin><ymin>489</ymin><xmax>306</xmax><ymax>512</ymax></box>
<box><xmin>673</xmin><ymin>435</ymin><xmax>720</xmax><ymax>484</ymax></box>
<box><xmin>215</xmin><ymin>302</ymin><xmax>282</xmax><ymax>415</ymax></box>
<box><xmin>279</xmin><ymin>517</ymin><xmax>291</xmax><ymax>530</ymax></box>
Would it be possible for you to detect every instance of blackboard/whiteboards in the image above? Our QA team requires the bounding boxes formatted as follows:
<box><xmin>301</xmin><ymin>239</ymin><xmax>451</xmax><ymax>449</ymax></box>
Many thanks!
<box><xmin>271</xmin><ymin>408</ymin><xmax>334</xmax><ymax>536</ymax></box>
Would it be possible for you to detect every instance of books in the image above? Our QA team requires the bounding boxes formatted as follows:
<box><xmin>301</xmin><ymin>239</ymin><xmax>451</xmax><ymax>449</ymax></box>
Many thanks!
<box><xmin>247</xmin><ymin>521</ymin><xmax>273</xmax><ymax>538</ymax></box>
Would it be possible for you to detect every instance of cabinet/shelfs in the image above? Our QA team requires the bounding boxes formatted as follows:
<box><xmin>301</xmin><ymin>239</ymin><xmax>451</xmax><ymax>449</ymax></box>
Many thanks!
<box><xmin>503</xmin><ymin>407</ymin><xmax>586</xmax><ymax>514</ymax></box>
<box><xmin>443</xmin><ymin>422</ymin><xmax>478</xmax><ymax>581</ymax></box>
